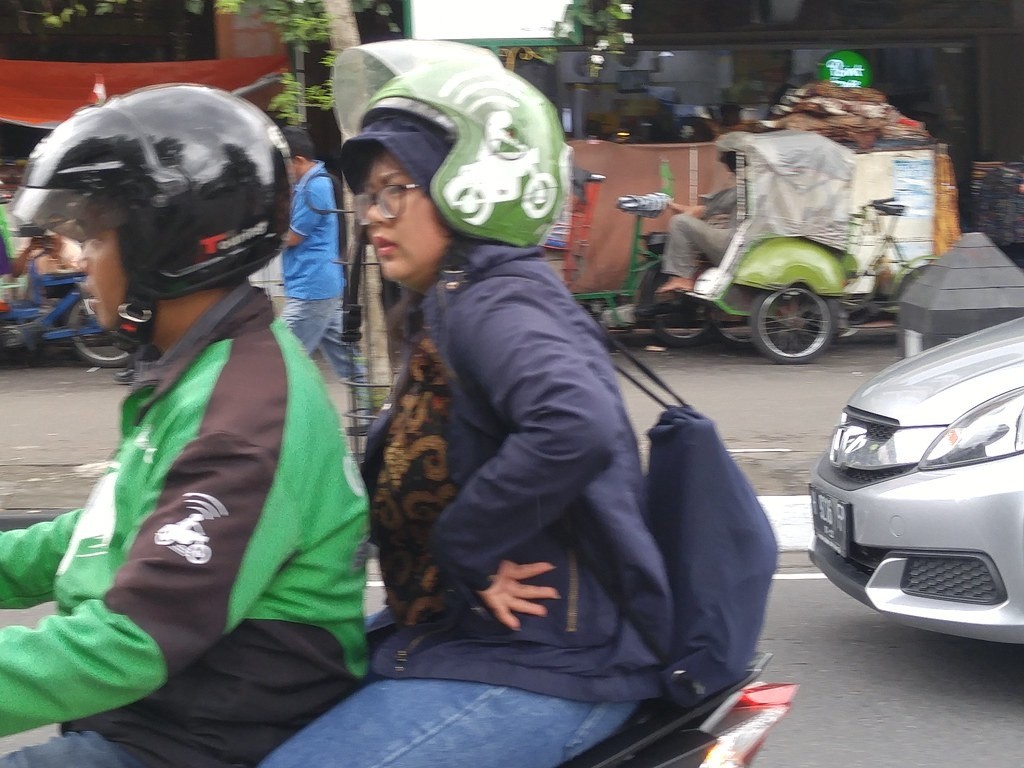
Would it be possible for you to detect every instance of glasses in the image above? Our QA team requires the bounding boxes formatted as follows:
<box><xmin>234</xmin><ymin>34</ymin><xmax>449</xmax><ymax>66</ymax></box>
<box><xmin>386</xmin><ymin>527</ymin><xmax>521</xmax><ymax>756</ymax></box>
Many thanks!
<box><xmin>350</xmin><ymin>180</ymin><xmax>420</xmax><ymax>227</ymax></box>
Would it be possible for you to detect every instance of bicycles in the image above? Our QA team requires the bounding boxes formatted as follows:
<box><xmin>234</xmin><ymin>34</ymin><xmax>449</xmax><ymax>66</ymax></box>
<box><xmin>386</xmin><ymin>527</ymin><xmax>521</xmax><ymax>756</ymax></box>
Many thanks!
<box><xmin>832</xmin><ymin>201</ymin><xmax>942</xmax><ymax>348</ymax></box>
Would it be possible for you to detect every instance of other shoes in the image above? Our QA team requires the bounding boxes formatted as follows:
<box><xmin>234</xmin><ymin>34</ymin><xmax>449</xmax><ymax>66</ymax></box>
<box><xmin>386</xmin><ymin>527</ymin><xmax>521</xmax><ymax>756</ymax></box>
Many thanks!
<box><xmin>113</xmin><ymin>365</ymin><xmax>135</xmax><ymax>384</ymax></box>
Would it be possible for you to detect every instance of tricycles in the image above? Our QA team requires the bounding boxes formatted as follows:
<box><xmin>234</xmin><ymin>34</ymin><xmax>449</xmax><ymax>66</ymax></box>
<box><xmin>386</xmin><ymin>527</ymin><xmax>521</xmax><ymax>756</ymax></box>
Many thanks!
<box><xmin>566</xmin><ymin>122</ymin><xmax>899</xmax><ymax>367</ymax></box>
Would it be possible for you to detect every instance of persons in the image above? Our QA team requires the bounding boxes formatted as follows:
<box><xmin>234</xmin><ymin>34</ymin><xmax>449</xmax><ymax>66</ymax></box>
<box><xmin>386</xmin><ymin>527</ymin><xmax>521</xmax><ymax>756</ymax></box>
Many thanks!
<box><xmin>12</xmin><ymin>214</ymin><xmax>82</xmax><ymax>278</ymax></box>
<box><xmin>654</xmin><ymin>151</ymin><xmax>737</xmax><ymax>294</ymax></box>
<box><xmin>0</xmin><ymin>82</ymin><xmax>371</xmax><ymax>768</ymax></box>
<box><xmin>254</xmin><ymin>38</ymin><xmax>673</xmax><ymax>768</ymax></box>
<box><xmin>277</xmin><ymin>127</ymin><xmax>366</xmax><ymax>394</ymax></box>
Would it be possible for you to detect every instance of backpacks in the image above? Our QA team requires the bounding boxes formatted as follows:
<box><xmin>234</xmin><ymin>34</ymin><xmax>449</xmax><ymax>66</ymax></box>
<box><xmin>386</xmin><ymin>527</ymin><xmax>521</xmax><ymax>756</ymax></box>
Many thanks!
<box><xmin>464</xmin><ymin>267</ymin><xmax>776</xmax><ymax>706</ymax></box>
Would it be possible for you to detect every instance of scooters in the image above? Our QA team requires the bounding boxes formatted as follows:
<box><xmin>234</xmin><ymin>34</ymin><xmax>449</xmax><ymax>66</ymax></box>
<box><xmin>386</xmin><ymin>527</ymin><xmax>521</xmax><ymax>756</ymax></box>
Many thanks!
<box><xmin>542</xmin><ymin>644</ymin><xmax>801</xmax><ymax>768</ymax></box>
<box><xmin>0</xmin><ymin>251</ymin><xmax>141</xmax><ymax>369</ymax></box>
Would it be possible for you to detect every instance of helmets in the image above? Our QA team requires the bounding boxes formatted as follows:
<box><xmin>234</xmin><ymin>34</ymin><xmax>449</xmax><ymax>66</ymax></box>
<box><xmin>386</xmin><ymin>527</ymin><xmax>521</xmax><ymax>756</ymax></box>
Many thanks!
<box><xmin>13</xmin><ymin>83</ymin><xmax>290</xmax><ymax>297</ymax></box>
<box><xmin>333</xmin><ymin>40</ymin><xmax>564</xmax><ymax>247</ymax></box>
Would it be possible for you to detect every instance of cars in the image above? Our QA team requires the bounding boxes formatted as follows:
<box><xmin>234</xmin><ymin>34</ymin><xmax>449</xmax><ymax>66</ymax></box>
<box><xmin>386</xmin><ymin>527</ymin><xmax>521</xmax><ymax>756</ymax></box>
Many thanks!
<box><xmin>805</xmin><ymin>315</ymin><xmax>1024</xmax><ymax>653</ymax></box>
